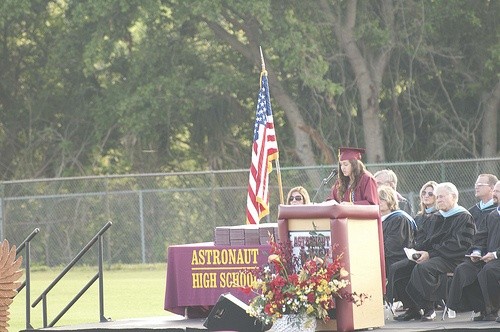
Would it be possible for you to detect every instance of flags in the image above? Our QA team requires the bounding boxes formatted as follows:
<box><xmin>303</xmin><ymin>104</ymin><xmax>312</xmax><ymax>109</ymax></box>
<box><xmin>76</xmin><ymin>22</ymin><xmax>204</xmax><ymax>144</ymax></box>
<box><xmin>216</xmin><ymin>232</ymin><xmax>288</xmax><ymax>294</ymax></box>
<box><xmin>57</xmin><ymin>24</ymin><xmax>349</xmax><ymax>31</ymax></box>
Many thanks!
<box><xmin>246</xmin><ymin>68</ymin><xmax>278</xmax><ymax>224</ymax></box>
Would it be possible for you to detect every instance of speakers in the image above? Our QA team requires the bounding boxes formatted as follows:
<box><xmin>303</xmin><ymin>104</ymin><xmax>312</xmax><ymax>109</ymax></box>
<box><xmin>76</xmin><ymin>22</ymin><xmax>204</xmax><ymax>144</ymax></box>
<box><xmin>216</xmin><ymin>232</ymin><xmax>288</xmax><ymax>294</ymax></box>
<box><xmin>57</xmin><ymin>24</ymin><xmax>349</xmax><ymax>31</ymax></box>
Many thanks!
<box><xmin>203</xmin><ymin>292</ymin><xmax>274</xmax><ymax>332</ymax></box>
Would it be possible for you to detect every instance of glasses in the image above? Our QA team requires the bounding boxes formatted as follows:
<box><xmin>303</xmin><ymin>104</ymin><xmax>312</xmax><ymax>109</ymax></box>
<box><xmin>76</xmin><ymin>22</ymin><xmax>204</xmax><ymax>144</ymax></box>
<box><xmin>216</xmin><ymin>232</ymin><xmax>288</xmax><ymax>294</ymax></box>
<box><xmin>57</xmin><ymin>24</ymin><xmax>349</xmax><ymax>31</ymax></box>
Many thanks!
<box><xmin>290</xmin><ymin>196</ymin><xmax>302</xmax><ymax>201</ymax></box>
<box><xmin>475</xmin><ymin>183</ymin><xmax>490</xmax><ymax>187</ymax></box>
<box><xmin>422</xmin><ymin>191</ymin><xmax>433</xmax><ymax>196</ymax></box>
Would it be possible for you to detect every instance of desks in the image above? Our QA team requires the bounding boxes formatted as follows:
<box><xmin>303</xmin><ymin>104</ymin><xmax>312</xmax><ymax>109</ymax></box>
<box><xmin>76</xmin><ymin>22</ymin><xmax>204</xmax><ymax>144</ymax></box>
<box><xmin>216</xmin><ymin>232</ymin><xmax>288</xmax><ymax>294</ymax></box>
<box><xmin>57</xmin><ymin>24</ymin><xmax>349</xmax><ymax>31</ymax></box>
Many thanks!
<box><xmin>164</xmin><ymin>242</ymin><xmax>273</xmax><ymax>321</ymax></box>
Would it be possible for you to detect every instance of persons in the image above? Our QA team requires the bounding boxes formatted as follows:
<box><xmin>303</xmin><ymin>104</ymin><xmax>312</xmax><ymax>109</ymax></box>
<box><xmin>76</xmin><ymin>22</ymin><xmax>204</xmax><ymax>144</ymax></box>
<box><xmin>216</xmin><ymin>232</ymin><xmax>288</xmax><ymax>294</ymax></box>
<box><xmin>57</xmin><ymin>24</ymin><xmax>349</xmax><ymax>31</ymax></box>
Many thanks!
<box><xmin>326</xmin><ymin>146</ymin><xmax>386</xmax><ymax>294</ymax></box>
<box><xmin>286</xmin><ymin>169</ymin><xmax>500</xmax><ymax>322</ymax></box>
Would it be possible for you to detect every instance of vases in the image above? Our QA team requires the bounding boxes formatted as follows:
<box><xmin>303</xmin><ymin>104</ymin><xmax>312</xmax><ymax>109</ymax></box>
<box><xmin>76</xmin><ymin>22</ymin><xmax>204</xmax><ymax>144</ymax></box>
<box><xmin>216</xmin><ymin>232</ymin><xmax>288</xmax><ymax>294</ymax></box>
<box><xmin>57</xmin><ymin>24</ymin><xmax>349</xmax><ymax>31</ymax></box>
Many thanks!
<box><xmin>265</xmin><ymin>314</ymin><xmax>318</xmax><ymax>332</ymax></box>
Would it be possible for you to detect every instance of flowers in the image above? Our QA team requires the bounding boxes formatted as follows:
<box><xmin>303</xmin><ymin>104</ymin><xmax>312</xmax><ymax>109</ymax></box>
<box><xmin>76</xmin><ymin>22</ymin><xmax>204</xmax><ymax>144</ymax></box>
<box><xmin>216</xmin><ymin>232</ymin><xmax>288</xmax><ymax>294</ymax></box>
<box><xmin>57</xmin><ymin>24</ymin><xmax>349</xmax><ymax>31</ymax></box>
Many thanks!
<box><xmin>223</xmin><ymin>220</ymin><xmax>372</xmax><ymax>329</ymax></box>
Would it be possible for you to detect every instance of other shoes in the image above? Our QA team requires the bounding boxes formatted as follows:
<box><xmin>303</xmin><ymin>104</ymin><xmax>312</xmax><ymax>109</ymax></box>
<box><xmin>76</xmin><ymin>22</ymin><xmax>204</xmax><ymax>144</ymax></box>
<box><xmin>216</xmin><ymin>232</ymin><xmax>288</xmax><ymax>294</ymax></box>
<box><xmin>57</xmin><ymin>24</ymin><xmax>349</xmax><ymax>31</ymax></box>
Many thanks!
<box><xmin>474</xmin><ymin>315</ymin><xmax>496</xmax><ymax>321</ymax></box>
<box><xmin>423</xmin><ymin>309</ymin><xmax>436</xmax><ymax>319</ymax></box>
<box><xmin>394</xmin><ymin>310</ymin><xmax>421</xmax><ymax>320</ymax></box>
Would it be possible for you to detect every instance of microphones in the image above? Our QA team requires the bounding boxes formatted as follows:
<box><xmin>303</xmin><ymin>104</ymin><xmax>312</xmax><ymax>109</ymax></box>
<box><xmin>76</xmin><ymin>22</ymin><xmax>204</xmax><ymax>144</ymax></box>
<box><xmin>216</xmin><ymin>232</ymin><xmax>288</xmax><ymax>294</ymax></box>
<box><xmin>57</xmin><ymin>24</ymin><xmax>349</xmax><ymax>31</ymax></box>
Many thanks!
<box><xmin>323</xmin><ymin>169</ymin><xmax>338</xmax><ymax>185</ymax></box>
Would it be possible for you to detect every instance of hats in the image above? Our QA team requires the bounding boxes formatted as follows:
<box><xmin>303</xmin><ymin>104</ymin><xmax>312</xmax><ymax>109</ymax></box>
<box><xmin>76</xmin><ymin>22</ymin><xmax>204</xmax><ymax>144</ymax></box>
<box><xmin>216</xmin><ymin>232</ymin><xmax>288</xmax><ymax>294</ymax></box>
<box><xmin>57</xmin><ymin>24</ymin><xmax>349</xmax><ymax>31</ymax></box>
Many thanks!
<box><xmin>339</xmin><ymin>147</ymin><xmax>364</xmax><ymax>160</ymax></box>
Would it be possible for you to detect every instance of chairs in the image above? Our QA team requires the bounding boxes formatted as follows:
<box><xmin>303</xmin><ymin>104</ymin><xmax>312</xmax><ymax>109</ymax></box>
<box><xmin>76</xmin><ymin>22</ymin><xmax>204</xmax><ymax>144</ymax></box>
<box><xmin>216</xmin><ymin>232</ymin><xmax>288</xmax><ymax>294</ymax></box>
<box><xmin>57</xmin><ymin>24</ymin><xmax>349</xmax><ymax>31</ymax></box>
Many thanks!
<box><xmin>386</xmin><ymin>258</ymin><xmax>413</xmax><ymax>321</ymax></box>
